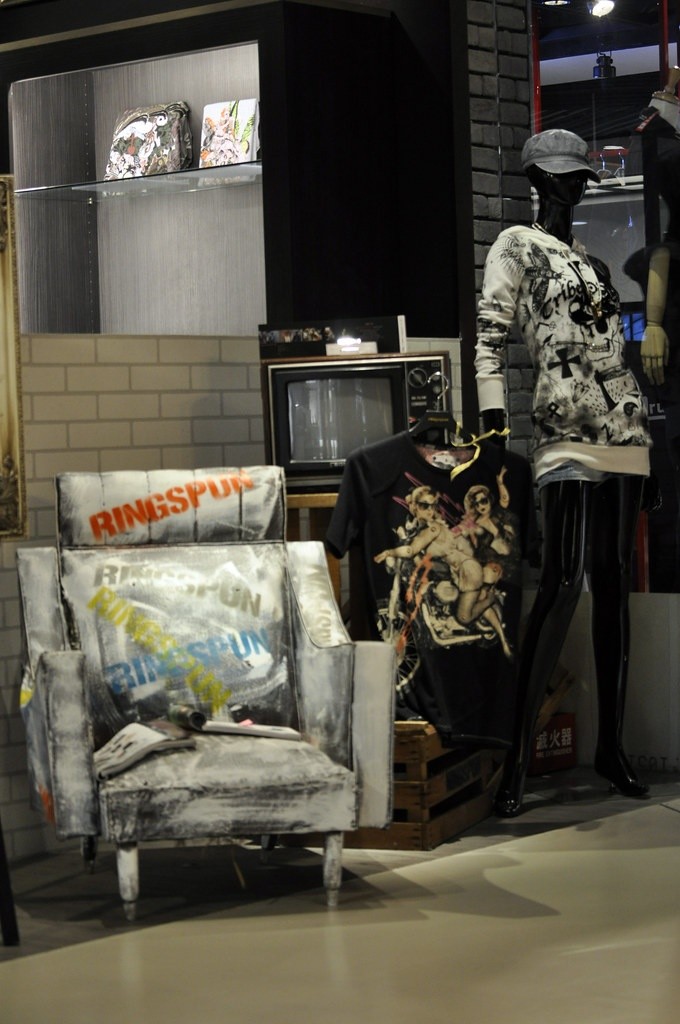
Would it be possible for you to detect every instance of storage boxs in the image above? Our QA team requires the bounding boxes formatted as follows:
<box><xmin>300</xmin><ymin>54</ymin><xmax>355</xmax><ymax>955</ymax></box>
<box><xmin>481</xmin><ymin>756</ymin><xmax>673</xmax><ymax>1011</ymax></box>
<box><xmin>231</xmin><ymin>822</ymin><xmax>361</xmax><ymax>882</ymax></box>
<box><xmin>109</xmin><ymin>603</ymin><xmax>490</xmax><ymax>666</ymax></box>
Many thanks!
<box><xmin>301</xmin><ymin>720</ymin><xmax>493</xmax><ymax>850</ymax></box>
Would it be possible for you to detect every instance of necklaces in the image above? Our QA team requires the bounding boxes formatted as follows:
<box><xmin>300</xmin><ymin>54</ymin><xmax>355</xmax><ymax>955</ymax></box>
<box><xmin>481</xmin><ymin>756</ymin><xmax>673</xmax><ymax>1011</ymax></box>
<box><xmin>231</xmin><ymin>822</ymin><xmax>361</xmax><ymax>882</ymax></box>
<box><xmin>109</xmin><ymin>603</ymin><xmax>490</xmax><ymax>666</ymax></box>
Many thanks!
<box><xmin>531</xmin><ymin>221</ymin><xmax>602</xmax><ymax>318</ymax></box>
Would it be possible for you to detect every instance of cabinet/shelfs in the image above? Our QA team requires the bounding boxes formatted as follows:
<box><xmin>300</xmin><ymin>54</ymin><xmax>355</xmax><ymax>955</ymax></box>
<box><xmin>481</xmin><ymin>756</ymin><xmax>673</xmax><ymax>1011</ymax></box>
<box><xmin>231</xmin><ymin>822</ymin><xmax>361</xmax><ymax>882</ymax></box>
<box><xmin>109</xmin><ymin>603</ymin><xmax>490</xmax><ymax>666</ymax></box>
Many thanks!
<box><xmin>7</xmin><ymin>39</ymin><xmax>268</xmax><ymax>338</ymax></box>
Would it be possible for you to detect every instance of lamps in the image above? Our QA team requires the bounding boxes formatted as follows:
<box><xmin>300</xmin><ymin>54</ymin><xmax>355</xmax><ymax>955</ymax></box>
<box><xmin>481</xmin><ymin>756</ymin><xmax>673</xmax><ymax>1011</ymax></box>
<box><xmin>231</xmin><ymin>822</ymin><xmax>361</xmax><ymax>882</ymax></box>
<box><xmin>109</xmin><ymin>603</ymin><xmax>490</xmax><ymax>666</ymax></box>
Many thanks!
<box><xmin>593</xmin><ymin>50</ymin><xmax>616</xmax><ymax>79</ymax></box>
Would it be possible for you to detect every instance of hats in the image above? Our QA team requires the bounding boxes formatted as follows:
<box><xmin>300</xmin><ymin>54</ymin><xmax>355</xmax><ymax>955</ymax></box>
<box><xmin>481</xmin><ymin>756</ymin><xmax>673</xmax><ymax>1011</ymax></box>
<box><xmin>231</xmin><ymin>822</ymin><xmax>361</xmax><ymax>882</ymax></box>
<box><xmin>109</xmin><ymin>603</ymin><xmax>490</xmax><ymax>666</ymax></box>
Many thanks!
<box><xmin>521</xmin><ymin>128</ymin><xmax>601</xmax><ymax>184</ymax></box>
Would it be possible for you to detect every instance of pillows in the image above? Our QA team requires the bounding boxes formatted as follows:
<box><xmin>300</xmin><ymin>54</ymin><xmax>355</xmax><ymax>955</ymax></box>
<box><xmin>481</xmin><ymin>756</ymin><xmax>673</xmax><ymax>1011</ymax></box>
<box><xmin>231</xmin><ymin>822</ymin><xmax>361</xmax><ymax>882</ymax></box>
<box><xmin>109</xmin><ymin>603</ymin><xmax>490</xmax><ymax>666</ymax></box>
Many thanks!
<box><xmin>59</xmin><ymin>548</ymin><xmax>302</xmax><ymax>732</ymax></box>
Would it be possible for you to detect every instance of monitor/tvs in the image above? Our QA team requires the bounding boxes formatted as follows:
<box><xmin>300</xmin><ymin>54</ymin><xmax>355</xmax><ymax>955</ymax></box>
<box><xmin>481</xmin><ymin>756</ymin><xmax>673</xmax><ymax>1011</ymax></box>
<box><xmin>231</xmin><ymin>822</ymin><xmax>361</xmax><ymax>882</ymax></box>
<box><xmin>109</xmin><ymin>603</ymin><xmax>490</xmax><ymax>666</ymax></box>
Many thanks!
<box><xmin>260</xmin><ymin>349</ymin><xmax>453</xmax><ymax>494</ymax></box>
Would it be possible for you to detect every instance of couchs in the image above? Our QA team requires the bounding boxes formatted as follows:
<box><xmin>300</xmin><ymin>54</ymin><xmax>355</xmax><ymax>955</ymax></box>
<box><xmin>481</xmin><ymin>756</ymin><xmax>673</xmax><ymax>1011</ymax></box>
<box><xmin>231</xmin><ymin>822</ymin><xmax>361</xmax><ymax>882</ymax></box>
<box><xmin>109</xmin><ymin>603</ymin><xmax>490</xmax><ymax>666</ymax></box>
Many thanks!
<box><xmin>15</xmin><ymin>464</ymin><xmax>395</xmax><ymax>926</ymax></box>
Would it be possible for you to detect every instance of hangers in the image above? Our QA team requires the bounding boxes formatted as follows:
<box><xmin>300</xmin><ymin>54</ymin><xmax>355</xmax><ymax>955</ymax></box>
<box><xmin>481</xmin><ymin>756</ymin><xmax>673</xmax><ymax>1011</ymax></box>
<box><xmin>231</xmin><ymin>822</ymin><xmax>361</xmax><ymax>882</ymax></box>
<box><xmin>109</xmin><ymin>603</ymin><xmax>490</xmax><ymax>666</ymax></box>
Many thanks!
<box><xmin>409</xmin><ymin>371</ymin><xmax>465</xmax><ymax>438</ymax></box>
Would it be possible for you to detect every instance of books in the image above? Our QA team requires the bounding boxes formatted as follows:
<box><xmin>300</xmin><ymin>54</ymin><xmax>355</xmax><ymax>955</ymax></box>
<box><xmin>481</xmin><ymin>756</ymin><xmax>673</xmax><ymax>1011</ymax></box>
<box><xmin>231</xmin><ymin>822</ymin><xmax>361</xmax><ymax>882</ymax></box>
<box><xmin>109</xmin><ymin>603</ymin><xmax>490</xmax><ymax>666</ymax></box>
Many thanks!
<box><xmin>92</xmin><ymin>719</ymin><xmax>197</xmax><ymax>783</ymax></box>
<box><xmin>168</xmin><ymin>704</ymin><xmax>301</xmax><ymax>741</ymax></box>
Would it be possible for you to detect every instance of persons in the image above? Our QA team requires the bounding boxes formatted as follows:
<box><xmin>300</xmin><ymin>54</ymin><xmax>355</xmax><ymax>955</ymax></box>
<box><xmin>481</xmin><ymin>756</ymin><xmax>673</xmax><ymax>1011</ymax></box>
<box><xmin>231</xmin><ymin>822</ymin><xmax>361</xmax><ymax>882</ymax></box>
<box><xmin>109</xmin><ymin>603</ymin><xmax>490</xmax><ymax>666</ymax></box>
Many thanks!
<box><xmin>258</xmin><ymin>327</ymin><xmax>336</xmax><ymax>344</ymax></box>
<box><xmin>472</xmin><ymin>129</ymin><xmax>651</xmax><ymax>820</ymax></box>
<box><xmin>622</xmin><ymin>244</ymin><xmax>680</xmax><ymax>503</ymax></box>
<box><xmin>640</xmin><ymin>65</ymin><xmax>680</xmax><ymax>257</ymax></box>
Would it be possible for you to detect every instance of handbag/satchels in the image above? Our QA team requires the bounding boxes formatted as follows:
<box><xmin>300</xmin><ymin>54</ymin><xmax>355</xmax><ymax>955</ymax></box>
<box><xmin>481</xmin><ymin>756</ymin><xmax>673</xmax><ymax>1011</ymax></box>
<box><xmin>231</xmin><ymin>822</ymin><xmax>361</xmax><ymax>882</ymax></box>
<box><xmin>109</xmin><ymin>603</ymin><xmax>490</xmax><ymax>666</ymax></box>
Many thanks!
<box><xmin>102</xmin><ymin>100</ymin><xmax>194</xmax><ymax>197</ymax></box>
<box><xmin>197</xmin><ymin>99</ymin><xmax>260</xmax><ymax>186</ymax></box>
